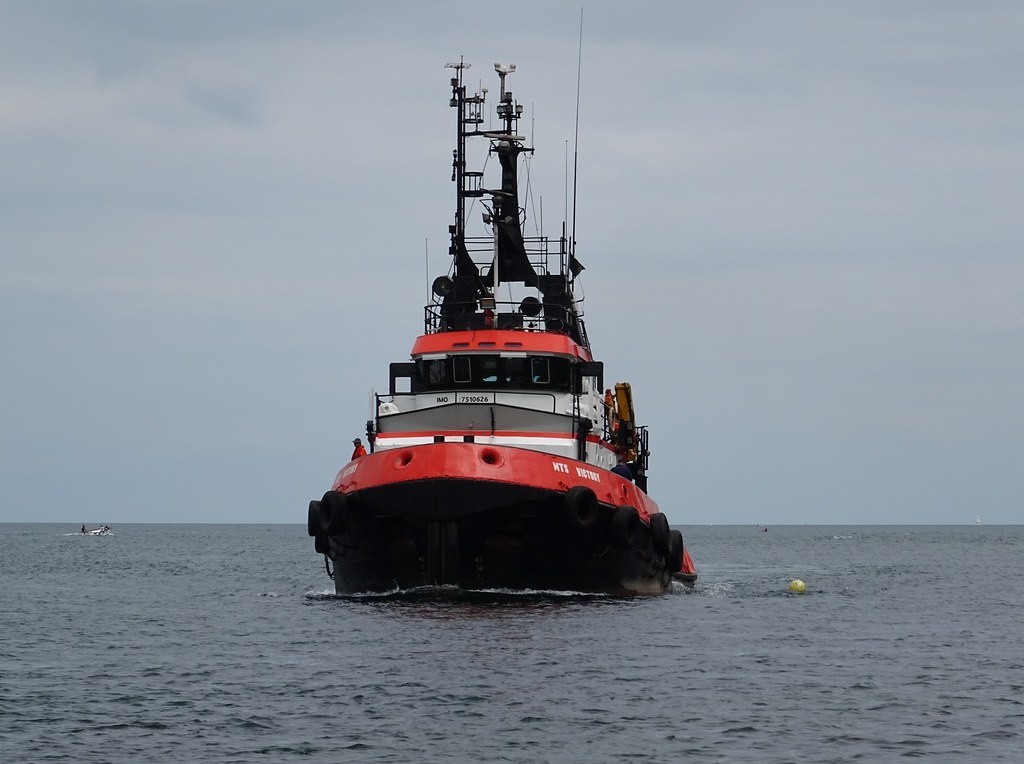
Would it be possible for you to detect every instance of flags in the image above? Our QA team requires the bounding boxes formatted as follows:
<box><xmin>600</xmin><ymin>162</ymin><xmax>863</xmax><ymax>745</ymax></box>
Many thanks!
<box><xmin>568</xmin><ymin>253</ymin><xmax>585</xmax><ymax>281</ymax></box>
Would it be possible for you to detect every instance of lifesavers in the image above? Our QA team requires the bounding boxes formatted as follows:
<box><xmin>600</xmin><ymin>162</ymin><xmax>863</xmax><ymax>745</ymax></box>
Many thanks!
<box><xmin>611</xmin><ymin>507</ymin><xmax>640</xmax><ymax>543</ymax></box>
<box><xmin>563</xmin><ymin>486</ymin><xmax>599</xmax><ymax>528</ymax></box>
<box><xmin>663</xmin><ymin>530</ymin><xmax>683</xmax><ymax>572</ymax></box>
<box><xmin>308</xmin><ymin>500</ymin><xmax>322</xmax><ymax>536</ymax></box>
<box><xmin>651</xmin><ymin>513</ymin><xmax>669</xmax><ymax>547</ymax></box>
<box><xmin>318</xmin><ymin>490</ymin><xmax>348</xmax><ymax>534</ymax></box>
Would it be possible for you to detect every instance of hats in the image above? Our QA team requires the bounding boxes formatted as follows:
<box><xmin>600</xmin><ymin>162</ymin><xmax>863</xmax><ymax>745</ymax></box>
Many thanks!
<box><xmin>352</xmin><ymin>437</ymin><xmax>361</xmax><ymax>442</ymax></box>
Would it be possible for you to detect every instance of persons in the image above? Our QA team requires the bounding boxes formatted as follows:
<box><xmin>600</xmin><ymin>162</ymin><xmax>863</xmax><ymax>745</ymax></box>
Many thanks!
<box><xmin>605</xmin><ymin>389</ymin><xmax>614</xmax><ymax>406</ymax></box>
<box><xmin>610</xmin><ymin>458</ymin><xmax>632</xmax><ymax>481</ymax></box>
<box><xmin>483</xmin><ymin>307</ymin><xmax>495</xmax><ymax>326</ymax></box>
<box><xmin>351</xmin><ymin>438</ymin><xmax>367</xmax><ymax>461</ymax></box>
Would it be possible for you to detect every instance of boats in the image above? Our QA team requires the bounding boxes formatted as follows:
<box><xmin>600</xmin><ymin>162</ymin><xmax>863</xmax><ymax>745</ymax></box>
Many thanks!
<box><xmin>308</xmin><ymin>53</ymin><xmax>697</xmax><ymax>602</ymax></box>
<box><xmin>703</xmin><ymin>522</ymin><xmax>712</xmax><ymax>526</ymax></box>
<box><xmin>757</xmin><ymin>522</ymin><xmax>765</xmax><ymax>527</ymax></box>
<box><xmin>81</xmin><ymin>527</ymin><xmax>110</xmax><ymax>536</ymax></box>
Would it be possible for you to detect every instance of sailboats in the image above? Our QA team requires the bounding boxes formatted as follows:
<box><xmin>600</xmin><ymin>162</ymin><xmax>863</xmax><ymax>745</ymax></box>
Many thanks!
<box><xmin>975</xmin><ymin>514</ymin><xmax>981</xmax><ymax>526</ymax></box>
